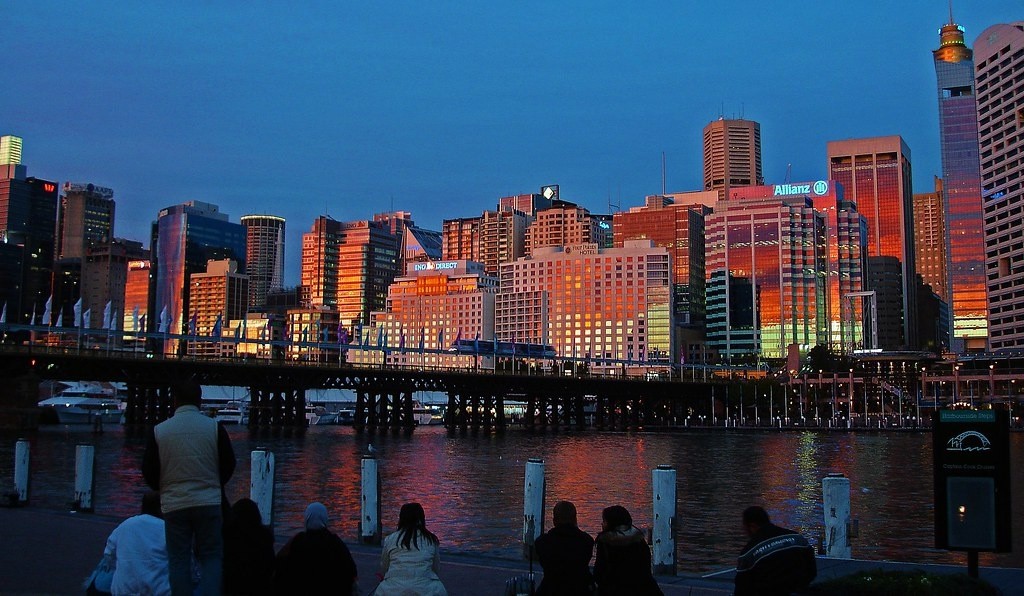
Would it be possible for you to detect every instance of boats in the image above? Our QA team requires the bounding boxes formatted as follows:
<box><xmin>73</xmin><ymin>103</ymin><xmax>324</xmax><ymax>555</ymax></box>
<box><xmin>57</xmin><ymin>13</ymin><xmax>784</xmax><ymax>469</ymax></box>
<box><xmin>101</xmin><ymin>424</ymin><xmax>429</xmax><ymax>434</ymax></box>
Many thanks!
<box><xmin>216</xmin><ymin>401</ymin><xmax>245</xmax><ymax>424</ymax></box>
<box><xmin>399</xmin><ymin>399</ymin><xmax>434</xmax><ymax>425</ymax></box>
<box><xmin>38</xmin><ymin>387</ymin><xmax>124</xmax><ymax>424</ymax></box>
<box><xmin>305</xmin><ymin>406</ymin><xmax>340</xmax><ymax>425</ymax></box>
<box><xmin>419</xmin><ymin>417</ymin><xmax>443</xmax><ymax>425</ymax></box>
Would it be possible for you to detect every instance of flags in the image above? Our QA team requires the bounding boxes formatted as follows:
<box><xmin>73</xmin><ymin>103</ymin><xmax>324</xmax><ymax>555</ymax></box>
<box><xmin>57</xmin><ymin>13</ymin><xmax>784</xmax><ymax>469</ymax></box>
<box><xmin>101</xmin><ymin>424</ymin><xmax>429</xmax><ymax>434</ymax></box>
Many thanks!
<box><xmin>539</xmin><ymin>340</ymin><xmax>707</xmax><ymax>365</ymax></box>
<box><xmin>439</xmin><ymin>329</ymin><xmax>444</xmax><ymax>352</ymax></box>
<box><xmin>419</xmin><ymin>327</ymin><xmax>425</xmax><ymax>354</ymax></box>
<box><xmin>399</xmin><ymin>325</ymin><xmax>408</xmax><ymax>354</ymax></box>
<box><xmin>235</xmin><ymin>313</ymin><xmax>246</xmax><ymax>344</ymax></box>
<box><xmin>512</xmin><ymin>333</ymin><xmax>515</xmax><ymax>354</ymax></box>
<box><xmin>303</xmin><ymin>319</ymin><xmax>328</xmax><ymax>344</ymax></box>
<box><xmin>454</xmin><ymin>331</ymin><xmax>462</xmax><ymax>353</ymax></box>
<box><xmin>378</xmin><ymin>324</ymin><xmax>389</xmax><ymax>351</ymax></box>
<box><xmin>0</xmin><ymin>294</ymin><xmax>173</xmax><ymax>338</ymax></box>
<box><xmin>338</xmin><ymin>323</ymin><xmax>348</xmax><ymax>353</ymax></box>
<box><xmin>190</xmin><ymin>309</ymin><xmax>198</xmax><ymax>335</ymax></box>
<box><xmin>357</xmin><ymin>322</ymin><xmax>369</xmax><ymax>350</ymax></box>
<box><xmin>474</xmin><ymin>332</ymin><xmax>479</xmax><ymax>352</ymax></box>
<box><xmin>494</xmin><ymin>333</ymin><xmax>498</xmax><ymax>354</ymax></box>
<box><xmin>212</xmin><ymin>311</ymin><xmax>222</xmax><ymax>344</ymax></box>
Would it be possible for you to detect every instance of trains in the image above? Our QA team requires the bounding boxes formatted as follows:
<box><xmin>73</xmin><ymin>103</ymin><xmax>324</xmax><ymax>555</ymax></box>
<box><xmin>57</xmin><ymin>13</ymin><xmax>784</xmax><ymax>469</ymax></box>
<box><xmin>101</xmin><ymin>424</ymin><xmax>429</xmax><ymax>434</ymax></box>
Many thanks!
<box><xmin>448</xmin><ymin>340</ymin><xmax>556</xmax><ymax>356</ymax></box>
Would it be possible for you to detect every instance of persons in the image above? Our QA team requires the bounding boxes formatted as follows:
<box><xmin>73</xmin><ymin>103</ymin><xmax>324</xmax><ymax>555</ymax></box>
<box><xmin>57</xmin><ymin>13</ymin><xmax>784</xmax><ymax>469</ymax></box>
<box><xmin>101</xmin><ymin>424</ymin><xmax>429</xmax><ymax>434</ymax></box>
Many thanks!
<box><xmin>734</xmin><ymin>506</ymin><xmax>817</xmax><ymax>596</ymax></box>
<box><xmin>523</xmin><ymin>502</ymin><xmax>665</xmax><ymax>596</ymax></box>
<box><xmin>98</xmin><ymin>376</ymin><xmax>360</xmax><ymax>596</ymax></box>
<box><xmin>370</xmin><ymin>503</ymin><xmax>448</xmax><ymax>596</ymax></box>
